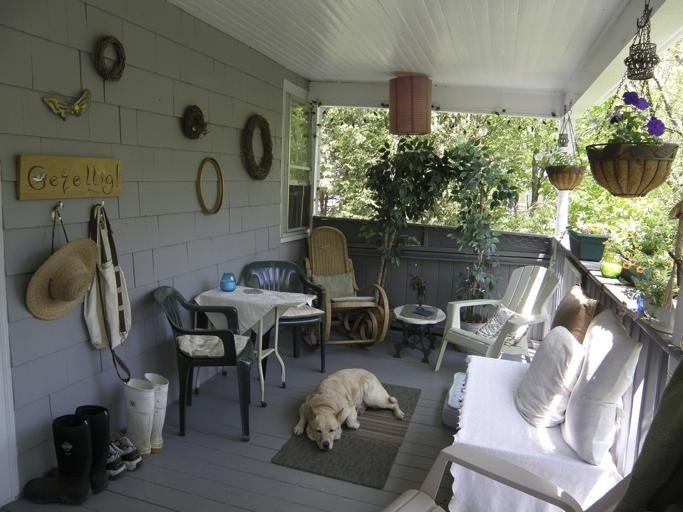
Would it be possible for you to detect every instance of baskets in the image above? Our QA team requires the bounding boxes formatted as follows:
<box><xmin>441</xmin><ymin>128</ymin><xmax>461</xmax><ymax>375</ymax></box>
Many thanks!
<box><xmin>545</xmin><ymin>164</ymin><xmax>587</xmax><ymax>191</ymax></box>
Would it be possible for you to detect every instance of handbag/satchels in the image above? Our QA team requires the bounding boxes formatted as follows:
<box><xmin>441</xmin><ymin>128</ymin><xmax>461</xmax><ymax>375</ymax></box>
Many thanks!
<box><xmin>84</xmin><ymin>208</ymin><xmax>131</xmax><ymax>350</ymax></box>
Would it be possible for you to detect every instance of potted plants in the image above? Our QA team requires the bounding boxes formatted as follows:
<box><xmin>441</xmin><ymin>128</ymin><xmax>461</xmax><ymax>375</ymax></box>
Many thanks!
<box><xmin>545</xmin><ymin>150</ymin><xmax>586</xmax><ymax>190</ymax></box>
<box><xmin>599</xmin><ymin>239</ymin><xmax>623</xmax><ymax>277</ymax></box>
<box><xmin>622</xmin><ymin>224</ymin><xmax>667</xmax><ymax>285</ymax></box>
<box><xmin>449</xmin><ymin>137</ymin><xmax>517</xmax><ymax>353</ymax></box>
<box><xmin>407</xmin><ymin>263</ymin><xmax>430</xmax><ymax>309</ymax></box>
<box><xmin>567</xmin><ymin>223</ymin><xmax>611</xmax><ymax>261</ymax></box>
<box><xmin>358</xmin><ymin>139</ymin><xmax>452</xmax><ymax>343</ymax></box>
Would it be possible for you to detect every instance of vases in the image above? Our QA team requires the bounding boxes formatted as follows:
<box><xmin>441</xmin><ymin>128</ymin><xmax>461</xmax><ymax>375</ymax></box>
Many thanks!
<box><xmin>645</xmin><ymin>298</ymin><xmax>662</xmax><ymax>317</ymax></box>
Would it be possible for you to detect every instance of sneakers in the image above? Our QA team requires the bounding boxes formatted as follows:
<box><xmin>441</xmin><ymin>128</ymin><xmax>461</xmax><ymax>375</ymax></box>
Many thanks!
<box><xmin>113</xmin><ymin>436</ymin><xmax>144</xmax><ymax>470</ymax></box>
<box><xmin>103</xmin><ymin>445</ymin><xmax>126</xmax><ymax>480</ymax></box>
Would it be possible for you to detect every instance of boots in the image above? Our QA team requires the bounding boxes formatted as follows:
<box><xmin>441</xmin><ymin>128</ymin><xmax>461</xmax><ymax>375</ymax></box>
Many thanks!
<box><xmin>123</xmin><ymin>379</ymin><xmax>154</xmax><ymax>455</ymax></box>
<box><xmin>76</xmin><ymin>405</ymin><xmax>110</xmax><ymax>494</ymax></box>
<box><xmin>23</xmin><ymin>413</ymin><xmax>90</xmax><ymax>505</ymax></box>
<box><xmin>144</xmin><ymin>373</ymin><xmax>170</xmax><ymax>454</ymax></box>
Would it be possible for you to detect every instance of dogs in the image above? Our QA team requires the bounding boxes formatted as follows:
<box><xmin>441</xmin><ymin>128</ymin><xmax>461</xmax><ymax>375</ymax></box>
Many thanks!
<box><xmin>293</xmin><ymin>368</ymin><xmax>405</xmax><ymax>451</ymax></box>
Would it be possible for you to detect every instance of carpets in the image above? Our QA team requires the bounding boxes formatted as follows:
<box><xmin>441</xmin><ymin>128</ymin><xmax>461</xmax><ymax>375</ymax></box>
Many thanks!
<box><xmin>270</xmin><ymin>383</ymin><xmax>421</xmax><ymax>490</ymax></box>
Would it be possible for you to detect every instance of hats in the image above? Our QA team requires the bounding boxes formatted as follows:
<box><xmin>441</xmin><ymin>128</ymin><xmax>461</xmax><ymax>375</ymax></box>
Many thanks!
<box><xmin>26</xmin><ymin>238</ymin><xmax>100</xmax><ymax>321</ymax></box>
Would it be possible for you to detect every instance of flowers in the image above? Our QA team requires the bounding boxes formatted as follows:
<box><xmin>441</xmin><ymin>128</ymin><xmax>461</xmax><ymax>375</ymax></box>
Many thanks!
<box><xmin>622</xmin><ymin>251</ymin><xmax>673</xmax><ymax>303</ymax></box>
<box><xmin>605</xmin><ymin>92</ymin><xmax>666</xmax><ymax>144</ymax></box>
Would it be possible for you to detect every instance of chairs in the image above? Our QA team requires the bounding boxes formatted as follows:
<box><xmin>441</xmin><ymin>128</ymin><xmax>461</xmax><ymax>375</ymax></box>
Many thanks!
<box><xmin>304</xmin><ymin>226</ymin><xmax>390</xmax><ymax>349</ymax></box>
<box><xmin>152</xmin><ymin>286</ymin><xmax>256</xmax><ymax>442</ymax></box>
<box><xmin>243</xmin><ymin>260</ymin><xmax>327</xmax><ymax>381</ymax></box>
<box><xmin>434</xmin><ymin>265</ymin><xmax>560</xmax><ymax>372</ymax></box>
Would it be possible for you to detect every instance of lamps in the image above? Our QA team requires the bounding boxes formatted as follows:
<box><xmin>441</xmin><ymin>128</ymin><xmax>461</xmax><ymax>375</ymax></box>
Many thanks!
<box><xmin>388</xmin><ymin>76</ymin><xmax>431</xmax><ymax>135</ymax></box>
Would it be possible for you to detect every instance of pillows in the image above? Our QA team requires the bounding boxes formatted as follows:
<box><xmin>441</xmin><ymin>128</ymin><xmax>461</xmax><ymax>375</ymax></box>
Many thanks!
<box><xmin>560</xmin><ymin>309</ymin><xmax>644</xmax><ymax>466</ymax></box>
<box><xmin>550</xmin><ymin>283</ymin><xmax>599</xmax><ymax>344</ymax></box>
<box><xmin>515</xmin><ymin>324</ymin><xmax>585</xmax><ymax>428</ymax></box>
<box><xmin>476</xmin><ymin>304</ymin><xmax>534</xmax><ymax>346</ymax></box>
